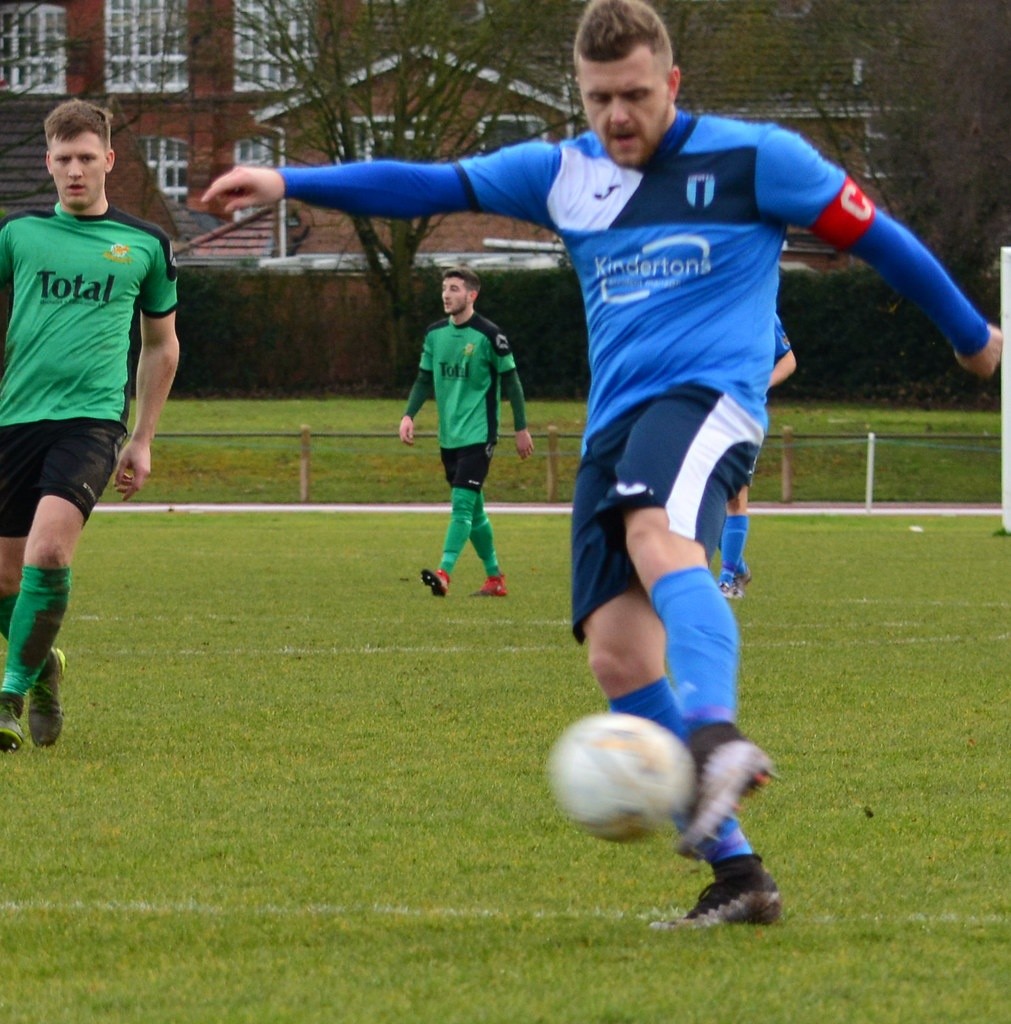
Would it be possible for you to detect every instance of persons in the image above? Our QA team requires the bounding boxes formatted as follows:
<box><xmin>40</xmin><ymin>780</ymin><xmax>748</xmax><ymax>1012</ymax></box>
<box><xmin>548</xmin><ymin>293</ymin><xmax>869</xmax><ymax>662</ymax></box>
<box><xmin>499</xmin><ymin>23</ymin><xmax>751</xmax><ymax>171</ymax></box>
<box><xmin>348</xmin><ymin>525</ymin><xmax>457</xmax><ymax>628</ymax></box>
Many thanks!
<box><xmin>0</xmin><ymin>99</ymin><xmax>180</xmax><ymax>750</ymax></box>
<box><xmin>207</xmin><ymin>3</ymin><xmax>999</xmax><ymax>929</ymax></box>
<box><xmin>398</xmin><ymin>263</ymin><xmax>532</xmax><ymax>603</ymax></box>
<box><xmin>713</xmin><ymin>311</ymin><xmax>799</xmax><ymax>598</ymax></box>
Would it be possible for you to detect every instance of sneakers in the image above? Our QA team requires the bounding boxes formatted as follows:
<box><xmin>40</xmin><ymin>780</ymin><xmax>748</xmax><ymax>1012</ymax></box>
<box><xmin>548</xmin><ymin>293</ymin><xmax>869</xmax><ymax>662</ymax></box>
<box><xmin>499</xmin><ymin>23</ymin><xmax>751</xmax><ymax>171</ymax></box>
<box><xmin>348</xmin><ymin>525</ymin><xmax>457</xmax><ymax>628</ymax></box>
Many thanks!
<box><xmin>474</xmin><ymin>573</ymin><xmax>506</xmax><ymax>596</ymax></box>
<box><xmin>716</xmin><ymin>568</ymin><xmax>751</xmax><ymax>600</ymax></box>
<box><xmin>422</xmin><ymin>568</ymin><xmax>448</xmax><ymax>596</ymax></box>
<box><xmin>0</xmin><ymin>691</ymin><xmax>25</xmax><ymax>750</ymax></box>
<box><xmin>678</xmin><ymin>738</ymin><xmax>773</xmax><ymax>851</ymax></box>
<box><xmin>27</xmin><ymin>647</ymin><xmax>69</xmax><ymax>746</ymax></box>
<box><xmin>654</xmin><ymin>880</ymin><xmax>779</xmax><ymax>928</ymax></box>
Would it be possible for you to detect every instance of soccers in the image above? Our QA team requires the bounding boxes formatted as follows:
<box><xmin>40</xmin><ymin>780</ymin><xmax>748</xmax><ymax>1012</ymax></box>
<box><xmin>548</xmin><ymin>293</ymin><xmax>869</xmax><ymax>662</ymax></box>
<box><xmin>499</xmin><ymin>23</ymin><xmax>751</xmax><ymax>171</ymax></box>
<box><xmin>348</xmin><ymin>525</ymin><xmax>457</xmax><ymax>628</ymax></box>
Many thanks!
<box><xmin>547</xmin><ymin>712</ymin><xmax>697</xmax><ymax>842</ymax></box>
